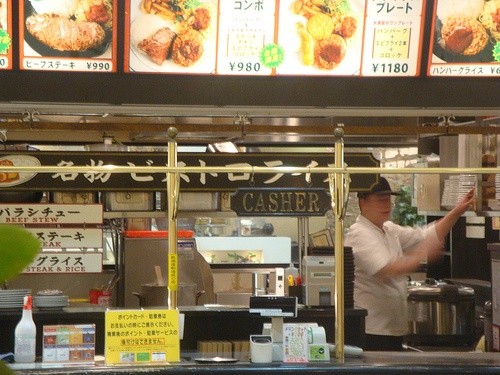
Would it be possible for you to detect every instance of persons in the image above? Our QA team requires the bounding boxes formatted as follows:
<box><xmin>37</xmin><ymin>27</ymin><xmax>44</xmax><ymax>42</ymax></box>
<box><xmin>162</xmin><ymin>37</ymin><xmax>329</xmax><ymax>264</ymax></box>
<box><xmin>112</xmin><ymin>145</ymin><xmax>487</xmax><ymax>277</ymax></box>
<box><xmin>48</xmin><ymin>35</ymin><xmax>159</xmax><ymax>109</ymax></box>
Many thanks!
<box><xmin>344</xmin><ymin>176</ymin><xmax>475</xmax><ymax>352</ymax></box>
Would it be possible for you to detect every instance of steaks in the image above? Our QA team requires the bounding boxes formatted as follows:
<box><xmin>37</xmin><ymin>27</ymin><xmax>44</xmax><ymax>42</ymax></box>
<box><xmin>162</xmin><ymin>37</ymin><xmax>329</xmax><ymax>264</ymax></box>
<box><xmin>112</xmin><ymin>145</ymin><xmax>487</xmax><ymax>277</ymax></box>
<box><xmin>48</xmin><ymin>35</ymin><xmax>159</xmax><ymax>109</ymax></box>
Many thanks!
<box><xmin>25</xmin><ymin>15</ymin><xmax>104</xmax><ymax>52</ymax></box>
<box><xmin>315</xmin><ymin>34</ymin><xmax>346</xmax><ymax>69</ymax></box>
<box><xmin>441</xmin><ymin>16</ymin><xmax>487</xmax><ymax>56</ymax></box>
<box><xmin>171</xmin><ymin>29</ymin><xmax>204</xmax><ymax>66</ymax></box>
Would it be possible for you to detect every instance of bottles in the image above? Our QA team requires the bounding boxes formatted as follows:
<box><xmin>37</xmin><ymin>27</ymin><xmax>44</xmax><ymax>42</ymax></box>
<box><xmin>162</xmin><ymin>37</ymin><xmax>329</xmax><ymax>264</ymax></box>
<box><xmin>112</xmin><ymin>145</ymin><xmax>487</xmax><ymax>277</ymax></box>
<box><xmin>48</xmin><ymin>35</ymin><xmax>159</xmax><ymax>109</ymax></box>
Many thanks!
<box><xmin>14</xmin><ymin>296</ymin><xmax>36</xmax><ymax>363</ymax></box>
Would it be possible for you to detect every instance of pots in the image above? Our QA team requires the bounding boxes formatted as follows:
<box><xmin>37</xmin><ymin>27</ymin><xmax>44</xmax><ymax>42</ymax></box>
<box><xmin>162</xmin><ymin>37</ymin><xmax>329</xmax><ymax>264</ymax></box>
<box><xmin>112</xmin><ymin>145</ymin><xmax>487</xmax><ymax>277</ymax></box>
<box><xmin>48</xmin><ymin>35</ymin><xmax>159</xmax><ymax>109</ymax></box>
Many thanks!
<box><xmin>406</xmin><ymin>283</ymin><xmax>476</xmax><ymax>347</ymax></box>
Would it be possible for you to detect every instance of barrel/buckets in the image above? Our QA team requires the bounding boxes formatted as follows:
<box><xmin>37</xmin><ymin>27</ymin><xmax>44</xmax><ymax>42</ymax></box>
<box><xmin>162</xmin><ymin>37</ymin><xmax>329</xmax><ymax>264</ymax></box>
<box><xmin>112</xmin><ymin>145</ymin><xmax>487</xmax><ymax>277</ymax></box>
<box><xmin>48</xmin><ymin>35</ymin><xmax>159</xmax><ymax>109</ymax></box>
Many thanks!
<box><xmin>89</xmin><ymin>289</ymin><xmax>112</xmax><ymax>306</ymax></box>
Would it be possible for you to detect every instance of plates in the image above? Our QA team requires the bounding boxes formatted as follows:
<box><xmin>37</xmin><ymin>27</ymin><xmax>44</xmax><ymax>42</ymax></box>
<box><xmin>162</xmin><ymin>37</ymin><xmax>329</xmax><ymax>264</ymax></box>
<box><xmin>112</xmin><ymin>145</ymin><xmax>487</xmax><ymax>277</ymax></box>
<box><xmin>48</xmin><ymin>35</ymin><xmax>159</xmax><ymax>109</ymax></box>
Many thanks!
<box><xmin>0</xmin><ymin>289</ymin><xmax>68</xmax><ymax>308</ymax></box>
<box><xmin>441</xmin><ymin>174</ymin><xmax>475</xmax><ymax>209</ymax></box>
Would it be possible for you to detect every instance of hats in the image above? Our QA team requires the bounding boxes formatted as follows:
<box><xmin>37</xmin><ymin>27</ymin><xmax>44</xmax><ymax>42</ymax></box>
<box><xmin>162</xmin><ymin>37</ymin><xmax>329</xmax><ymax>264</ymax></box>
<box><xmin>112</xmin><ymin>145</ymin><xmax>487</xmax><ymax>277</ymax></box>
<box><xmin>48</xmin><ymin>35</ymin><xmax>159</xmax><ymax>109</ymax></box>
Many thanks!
<box><xmin>357</xmin><ymin>177</ymin><xmax>403</xmax><ymax>197</ymax></box>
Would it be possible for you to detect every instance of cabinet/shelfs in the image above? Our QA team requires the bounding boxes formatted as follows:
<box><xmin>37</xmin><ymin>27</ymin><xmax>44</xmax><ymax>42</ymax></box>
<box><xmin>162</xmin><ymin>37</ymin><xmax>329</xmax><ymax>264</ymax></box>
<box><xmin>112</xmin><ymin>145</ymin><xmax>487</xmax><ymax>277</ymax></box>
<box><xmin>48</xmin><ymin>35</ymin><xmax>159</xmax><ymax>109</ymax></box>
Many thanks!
<box><xmin>417</xmin><ymin>210</ymin><xmax>500</xmax><ymax>284</ymax></box>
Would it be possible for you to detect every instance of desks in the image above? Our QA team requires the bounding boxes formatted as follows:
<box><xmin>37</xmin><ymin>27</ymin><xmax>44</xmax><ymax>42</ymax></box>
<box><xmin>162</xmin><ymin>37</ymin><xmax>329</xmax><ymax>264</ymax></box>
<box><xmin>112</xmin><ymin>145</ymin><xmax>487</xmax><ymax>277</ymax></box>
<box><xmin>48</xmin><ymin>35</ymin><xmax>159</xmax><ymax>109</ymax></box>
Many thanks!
<box><xmin>0</xmin><ymin>351</ymin><xmax>500</xmax><ymax>375</ymax></box>
<box><xmin>0</xmin><ymin>304</ymin><xmax>369</xmax><ymax>351</ymax></box>
